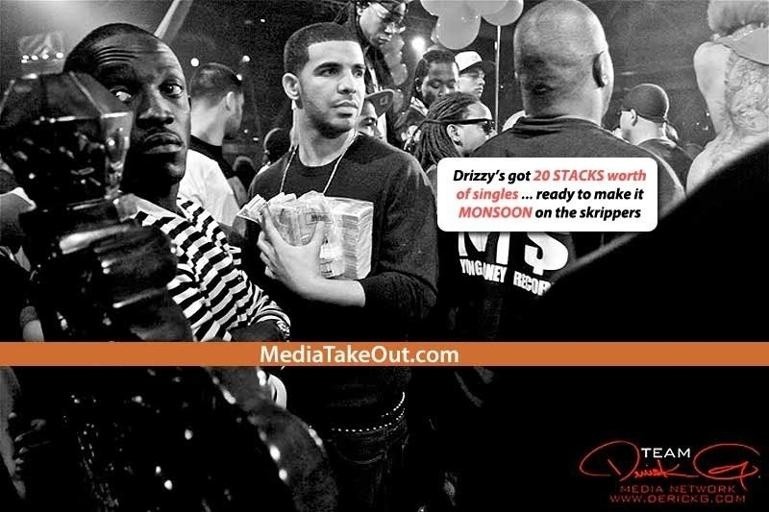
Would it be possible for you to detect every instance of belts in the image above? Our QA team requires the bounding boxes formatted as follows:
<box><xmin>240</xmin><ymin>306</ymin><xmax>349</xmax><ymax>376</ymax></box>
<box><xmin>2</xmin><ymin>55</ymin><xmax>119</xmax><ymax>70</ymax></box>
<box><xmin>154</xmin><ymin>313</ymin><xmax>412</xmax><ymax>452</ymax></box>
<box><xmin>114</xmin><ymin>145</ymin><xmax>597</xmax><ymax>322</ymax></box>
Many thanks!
<box><xmin>324</xmin><ymin>391</ymin><xmax>408</xmax><ymax>436</ymax></box>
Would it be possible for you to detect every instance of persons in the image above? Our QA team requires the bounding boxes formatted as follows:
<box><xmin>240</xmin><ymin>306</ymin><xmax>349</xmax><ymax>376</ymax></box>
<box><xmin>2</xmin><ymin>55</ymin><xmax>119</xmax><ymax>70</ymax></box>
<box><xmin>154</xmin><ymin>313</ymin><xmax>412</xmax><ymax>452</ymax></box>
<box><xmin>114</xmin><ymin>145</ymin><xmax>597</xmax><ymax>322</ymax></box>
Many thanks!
<box><xmin>0</xmin><ymin>0</ymin><xmax>769</xmax><ymax>512</ymax></box>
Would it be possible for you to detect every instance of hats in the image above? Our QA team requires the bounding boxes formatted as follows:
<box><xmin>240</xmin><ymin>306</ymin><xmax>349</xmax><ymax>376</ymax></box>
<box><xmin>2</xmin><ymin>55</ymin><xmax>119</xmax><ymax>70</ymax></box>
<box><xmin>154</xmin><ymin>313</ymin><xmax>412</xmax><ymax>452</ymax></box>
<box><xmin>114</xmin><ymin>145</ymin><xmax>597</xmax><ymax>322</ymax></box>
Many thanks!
<box><xmin>620</xmin><ymin>82</ymin><xmax>671</xmax><ymax>123</ymax></box>
<box><xmin>454</xmin><ymin>51</ymin><xmax>497</xmax><ymax>76</ymax></box>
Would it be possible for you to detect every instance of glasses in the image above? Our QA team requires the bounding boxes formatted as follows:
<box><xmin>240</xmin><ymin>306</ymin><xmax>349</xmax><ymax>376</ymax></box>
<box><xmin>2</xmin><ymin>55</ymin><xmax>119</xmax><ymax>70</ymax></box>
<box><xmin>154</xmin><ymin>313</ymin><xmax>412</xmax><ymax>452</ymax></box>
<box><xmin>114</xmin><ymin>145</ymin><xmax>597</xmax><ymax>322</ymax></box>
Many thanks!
<box><xmin>367</xmin><ymin>2</ymin><xmax>406</xmax><ymax>34</ymax></box>
<box><xmin>449</xmin><ymin>116</ymin><xmax>497</xmax><ymax>134</ymax></box>
